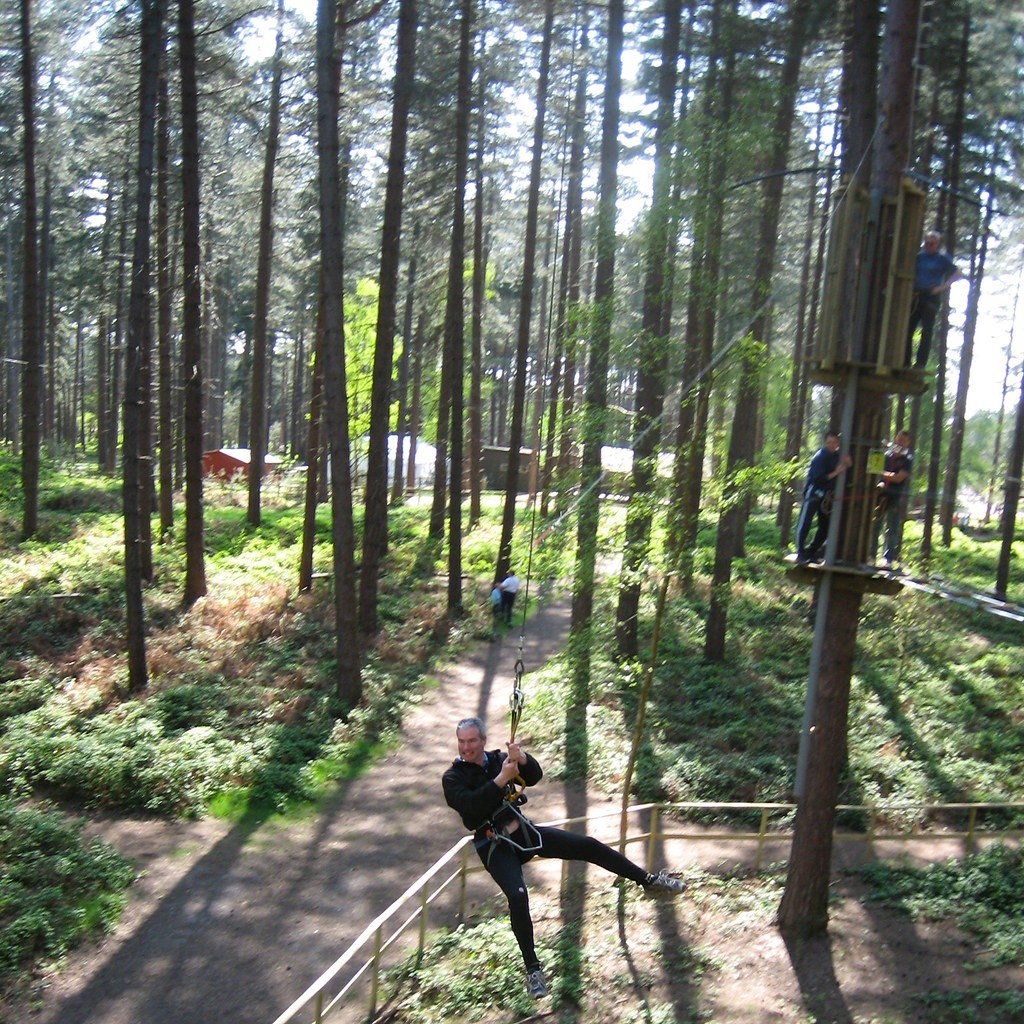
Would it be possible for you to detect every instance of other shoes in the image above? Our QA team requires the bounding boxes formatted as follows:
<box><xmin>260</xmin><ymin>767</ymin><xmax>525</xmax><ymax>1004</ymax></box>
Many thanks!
<box><xmin>795</xmin><ymin>551</ymin><xmax>811</xmax><ymax>564</ymax></box>
<box><xmin>878</xmin><ymin>566</ymin><xmax>891</xmax><ymax>570</ymax></box>
<box><xmin>914</xmin><ymin>362</ymin><xmax>925</xmax><ymax>370</ymax></box>
<box><xmin>806</xmin><ymin>549</ymin><xmax>820</xmax><ymax>564</ymax></box>
<box><xmin>903</xmin><ymin>362</ymin><xmax>912</xmax><ymax>370</ymax></box>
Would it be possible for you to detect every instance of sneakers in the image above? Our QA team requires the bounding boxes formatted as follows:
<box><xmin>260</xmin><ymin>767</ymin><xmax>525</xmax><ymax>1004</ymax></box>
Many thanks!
<box><xmin>644</xmin><ymin>872</ymin><xmax>686</xmax><ymax>896</ymax></box>
<box><xmin>525</xmin><ymin>969</ymin><xmax>547</xmax><ymax>1000</ymax></box>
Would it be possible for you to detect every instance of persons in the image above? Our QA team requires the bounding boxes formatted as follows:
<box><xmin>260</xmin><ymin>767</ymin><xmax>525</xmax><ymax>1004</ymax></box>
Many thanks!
<box><xmin>874</xmin><ymin>428</ymin><xmax>912</xmax><ymax>573</ymax></box>
<box><xmin>442</xmin><ymin>717</ymin><xmax>687</xmax><ymax>1001</ymax></box>
<box><xmin>796</xmin><ymin>431</ymin><xmax>853</xmax><ymax>567</ymax></box>
<box><xmin>901</xmin><ymin>232</ymin><xmax>961</xmax><ymax>362</ymax></box>
<box><xmin>489</xmin><ymin>567</ymin><xmax>518</xmax><ymax>627</ymax></box>
